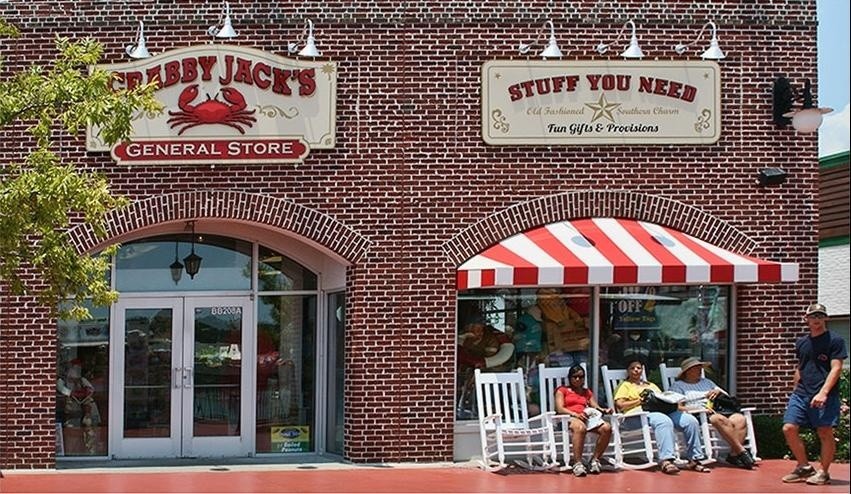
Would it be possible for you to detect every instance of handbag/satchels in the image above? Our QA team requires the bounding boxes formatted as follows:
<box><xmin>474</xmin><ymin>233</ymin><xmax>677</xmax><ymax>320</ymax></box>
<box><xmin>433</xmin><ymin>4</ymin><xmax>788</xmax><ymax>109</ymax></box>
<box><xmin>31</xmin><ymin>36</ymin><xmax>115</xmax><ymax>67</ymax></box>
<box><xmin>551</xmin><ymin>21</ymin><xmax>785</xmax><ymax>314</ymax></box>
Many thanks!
<box><xmin>582</xmin><ymin>407</ymin><xmax>605</xmax><ymax>433</ymax></box>
<box><xmin>712</xmin><ymin>392</ymin><xmax>741</xmax><ymax>418</ymax></box>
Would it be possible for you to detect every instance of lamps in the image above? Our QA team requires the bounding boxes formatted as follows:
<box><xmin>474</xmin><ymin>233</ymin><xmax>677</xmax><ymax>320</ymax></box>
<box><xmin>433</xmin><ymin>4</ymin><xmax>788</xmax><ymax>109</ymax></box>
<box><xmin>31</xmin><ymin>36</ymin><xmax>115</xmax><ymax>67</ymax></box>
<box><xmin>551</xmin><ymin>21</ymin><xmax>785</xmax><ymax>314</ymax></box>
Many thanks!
<box><xmin>675</xmin><ymin>19</ymin><xmax>724</xmax><ymax>60</ymax></box>
<box><xmin>757</xmin><ymin>166</ymin><xmax>787</xmax><ymax>188</ymax></box>
<box><xmin>768</xmin><ymin>72</ymin><xmax>833</xmax><ymax>134</ymax></box>
<box><xmin>122</xmin><ymin>17</ymin><xmax>153</xmax><ymax>63</ymax></box>
<box><xmin>206</xmin><ymin>0</ymin><xmax>244</xmax><ymax>39</ymax></box>
<box><xmin>595</xmin><ymin>19</ymin><xmax>645</xmax><ymax>59</ymax></box>
<box><xmin>519</xmin><ymin>19</ymin><xmax>566</xmax><ymax>60</ymax></box>
<box><xmin>184</xmin><ymin>218</ymin><xmax>201</xmax><ymax>279</ymax></box>
<box><xmin>284</xmin><ymin>18</ymin><xmax>323</xmax><ymax>62</ymax></box>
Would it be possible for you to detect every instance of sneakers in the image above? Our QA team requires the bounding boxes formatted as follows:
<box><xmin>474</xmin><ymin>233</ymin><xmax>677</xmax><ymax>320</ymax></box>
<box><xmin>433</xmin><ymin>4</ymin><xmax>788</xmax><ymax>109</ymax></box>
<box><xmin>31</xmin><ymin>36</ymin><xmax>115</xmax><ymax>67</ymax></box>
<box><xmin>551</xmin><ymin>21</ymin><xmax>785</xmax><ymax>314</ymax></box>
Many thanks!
<box><xmin>660</xmin><ymin>460</ymin><xmax>679</xmax><ymax>474</ymax></box>
<box><xmin>782</xmin><ymin>464</ymin><xmax>818</xmax><ymax>483</ymax></box>
<box><xmin>806</xmin><ymin>469</ymin><xmax>832</xmax><ymax>486</ymax></box>
<box><xmin>573</xmin><ymin>462</ymin><xmax>588</xmax><ymax>477</ymax></box>
<box><xmin>688</xmin><ymin>460</ymin><xmax>711</xmax><ymax>473</ymax></box>
<box><xmin>725</xmin><ymin>449</ymin><xmax>755</xmax><ymax>470</ymax></box>
<box><xmin>588</xmin><ymin>456</ymin><xmax>602</xmax><ymax>474</ymax></box>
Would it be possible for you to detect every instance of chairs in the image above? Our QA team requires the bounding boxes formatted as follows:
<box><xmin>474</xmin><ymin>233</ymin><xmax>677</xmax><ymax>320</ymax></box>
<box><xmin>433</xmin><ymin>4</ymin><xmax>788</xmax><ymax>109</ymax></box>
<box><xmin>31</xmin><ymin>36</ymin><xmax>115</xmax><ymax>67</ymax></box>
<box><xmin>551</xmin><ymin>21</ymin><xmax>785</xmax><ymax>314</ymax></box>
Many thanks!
<box><xmin>602</xmin><ymin>363</ymin><xmax>690</xmax><ymax>470</ymax></box>
<box><xmin>534</xmin><ymin>363</ymin><xmax>611</xmax><ymax>473</ymax></box>
<box><xmin>472</xmin><ymin>366</ymin><xmax>560</xmax><ymax>474</ymax></box>
<box><xmin>660</xmin><ymin>361</ymin><xmax>762</xmax><ymax>467</ymax></box>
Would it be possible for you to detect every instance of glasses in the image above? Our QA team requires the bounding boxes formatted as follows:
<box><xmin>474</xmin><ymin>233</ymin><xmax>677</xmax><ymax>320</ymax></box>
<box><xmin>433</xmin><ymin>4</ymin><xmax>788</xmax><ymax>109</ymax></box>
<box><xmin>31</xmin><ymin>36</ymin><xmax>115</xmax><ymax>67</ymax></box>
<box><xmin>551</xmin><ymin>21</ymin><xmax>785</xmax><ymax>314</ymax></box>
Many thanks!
<box><xmin>810</xmin><ymin>313</ymin><xmax>825</xmax><ymax>319</ymax></box>
<box><xmin>571</xmin><ymin>375</ymin><xmax>584</xmax><ymax>380</ymax></box>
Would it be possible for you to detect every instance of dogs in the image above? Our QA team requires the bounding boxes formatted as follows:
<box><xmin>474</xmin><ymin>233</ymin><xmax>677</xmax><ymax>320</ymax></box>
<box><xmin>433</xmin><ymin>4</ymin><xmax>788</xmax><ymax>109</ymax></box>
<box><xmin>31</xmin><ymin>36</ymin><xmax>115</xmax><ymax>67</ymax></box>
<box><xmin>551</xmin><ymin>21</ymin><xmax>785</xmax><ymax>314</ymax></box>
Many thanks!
<box><xmin>706</xmin><ymin>391</ymin><xmax>741</xmax><ymax>412</ymax></box>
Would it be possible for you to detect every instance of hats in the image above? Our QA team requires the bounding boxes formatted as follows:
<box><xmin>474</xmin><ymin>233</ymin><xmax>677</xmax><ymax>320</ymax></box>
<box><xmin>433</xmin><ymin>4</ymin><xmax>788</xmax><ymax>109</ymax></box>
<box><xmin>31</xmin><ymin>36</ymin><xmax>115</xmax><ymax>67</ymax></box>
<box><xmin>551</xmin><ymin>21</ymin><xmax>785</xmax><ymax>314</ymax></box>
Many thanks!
<box><xmin>805</xmin><ymin>304</ymin><xmax>827</xmax><ymax>317</ymax></box>
<box><xmin>677</xmin><ymin>357</ymin><xmax>712</xmax><ymax>378</ymax></box>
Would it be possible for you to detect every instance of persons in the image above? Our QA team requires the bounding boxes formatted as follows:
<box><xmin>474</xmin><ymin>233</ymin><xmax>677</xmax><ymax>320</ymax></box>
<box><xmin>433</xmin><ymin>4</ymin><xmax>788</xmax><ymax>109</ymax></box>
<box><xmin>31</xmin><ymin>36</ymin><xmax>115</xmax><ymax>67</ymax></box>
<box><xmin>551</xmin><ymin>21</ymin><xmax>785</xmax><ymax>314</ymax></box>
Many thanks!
<box><xmin>553</xmin><ymin>362</ymin><xmax>611</xmax><ymax>478</ymax></box>
<box><xmin>668</xmin><ymin>356</ymin><xmax>758</xmax><ymax>470</ymax></box>
<box><xmin>57</xmin><ymin>359</ymin><xmax>96</xmax><ymax>428</ymax></box>
<box><xmin>614</xmin><ymin>361</ymin><xmax>712</xmax><ymax>475</ymax></box>
<box><xmin>780</xmin><ymin>304</ymin><xmax>849</xmax><ymax>486</ymax></box>
<box><xmin>512</xmin><ymin>306</ymin><xmax>545</xmax><ymax>372</ymax></box>
